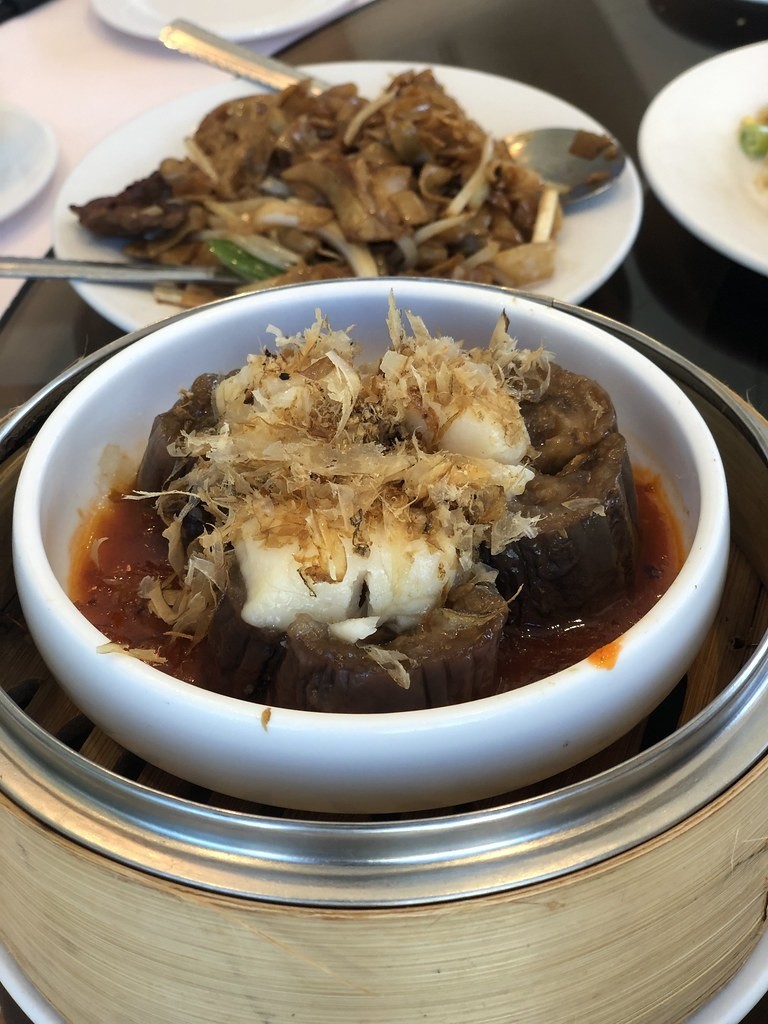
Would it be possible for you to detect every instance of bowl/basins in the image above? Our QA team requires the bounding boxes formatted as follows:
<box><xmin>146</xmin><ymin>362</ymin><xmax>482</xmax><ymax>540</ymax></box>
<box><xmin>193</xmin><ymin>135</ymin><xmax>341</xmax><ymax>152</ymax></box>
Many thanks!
<box><xmin>10</xmin><ymin>279</ymin><xmax>730</xmax><ymax>818</ymax></box>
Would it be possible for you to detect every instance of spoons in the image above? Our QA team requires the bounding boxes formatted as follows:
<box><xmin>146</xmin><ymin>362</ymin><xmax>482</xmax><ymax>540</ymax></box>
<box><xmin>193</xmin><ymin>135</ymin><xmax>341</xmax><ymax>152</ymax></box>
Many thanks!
<box><xmin>159</xmin><ymin>19</ymin><xmax>626</xmax><ymax>203</ymax></box>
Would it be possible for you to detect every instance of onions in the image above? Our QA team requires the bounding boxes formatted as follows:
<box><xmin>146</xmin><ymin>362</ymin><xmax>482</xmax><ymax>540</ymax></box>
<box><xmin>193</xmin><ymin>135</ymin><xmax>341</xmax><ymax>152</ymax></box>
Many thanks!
<box><xmin>137</xmin><ymin>92</ymin><xmax>559</xmax><ymax>307</ymax></box>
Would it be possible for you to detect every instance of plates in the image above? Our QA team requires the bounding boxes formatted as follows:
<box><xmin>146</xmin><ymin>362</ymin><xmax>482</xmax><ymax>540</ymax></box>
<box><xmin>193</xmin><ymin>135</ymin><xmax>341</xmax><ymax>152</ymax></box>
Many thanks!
<box><xmin>636</xmin><ymin>42</ymin><xmax>768</xmax><ymax>276</ymax></box>
<box><xmin>0</xmin><ymin>105</ymin><xmax>57</xmax><ymax>225</ymax></box>
<box><xmin>48</xmin><ymin>66</ymin><xmax>642</xmax><ymax>335</ymax></box>
<box><xmin>89</xmin><ymin>0</ymin><xmax>358</xmax><ymax>43</ymax></box>
